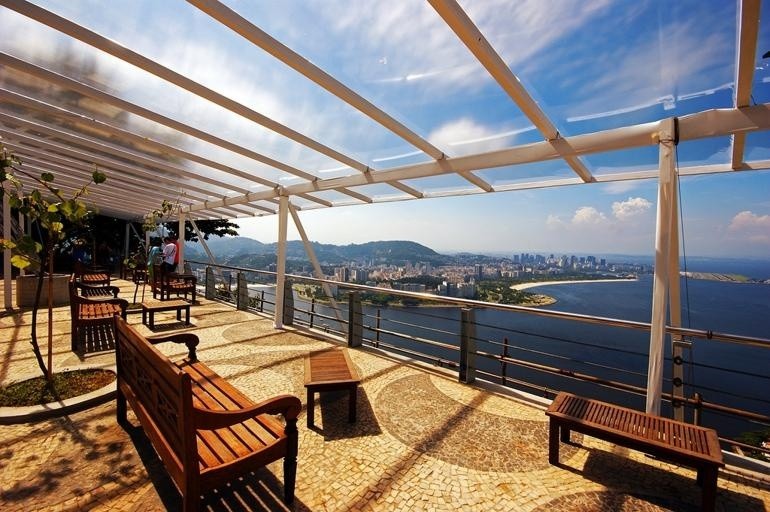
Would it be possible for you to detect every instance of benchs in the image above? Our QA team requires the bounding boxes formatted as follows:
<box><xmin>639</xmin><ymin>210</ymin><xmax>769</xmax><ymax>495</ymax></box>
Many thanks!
<box><xmin>69</xmin><ymin>257</ymin><xmax>129</xmax><ymax>350</ymax></box>
<box><xmin>152</xmin><ymin>264</ymin><xmax>197</xmax><ymax>305</ymax></box>
<box><xmin>107</xmin><ymin>311</ymin><xmax>301</xmax><ymax>511</ymax></box>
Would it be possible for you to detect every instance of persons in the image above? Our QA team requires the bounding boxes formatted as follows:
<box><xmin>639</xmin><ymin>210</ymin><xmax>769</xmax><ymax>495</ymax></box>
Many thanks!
<box><xmin>133</xmin><ymin>234</ymin><xmax>179</xmax><ymax>294</ymax></box>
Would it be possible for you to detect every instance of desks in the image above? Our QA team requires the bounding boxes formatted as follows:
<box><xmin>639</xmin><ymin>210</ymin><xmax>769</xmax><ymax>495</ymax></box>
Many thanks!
<box><xmin>546</xmin><ymin>390</ymin><xmax>724</xmax><ymax>507</ymax></box>
<box><xmin>302</xmin><ymin>345</ymin><xmax>362</xmax><ymax>429</ymax></box>
<box><xmin>141</xmin><ymin>299</ymin><xmax>192</xmax><ymax>329</ymax></box>
